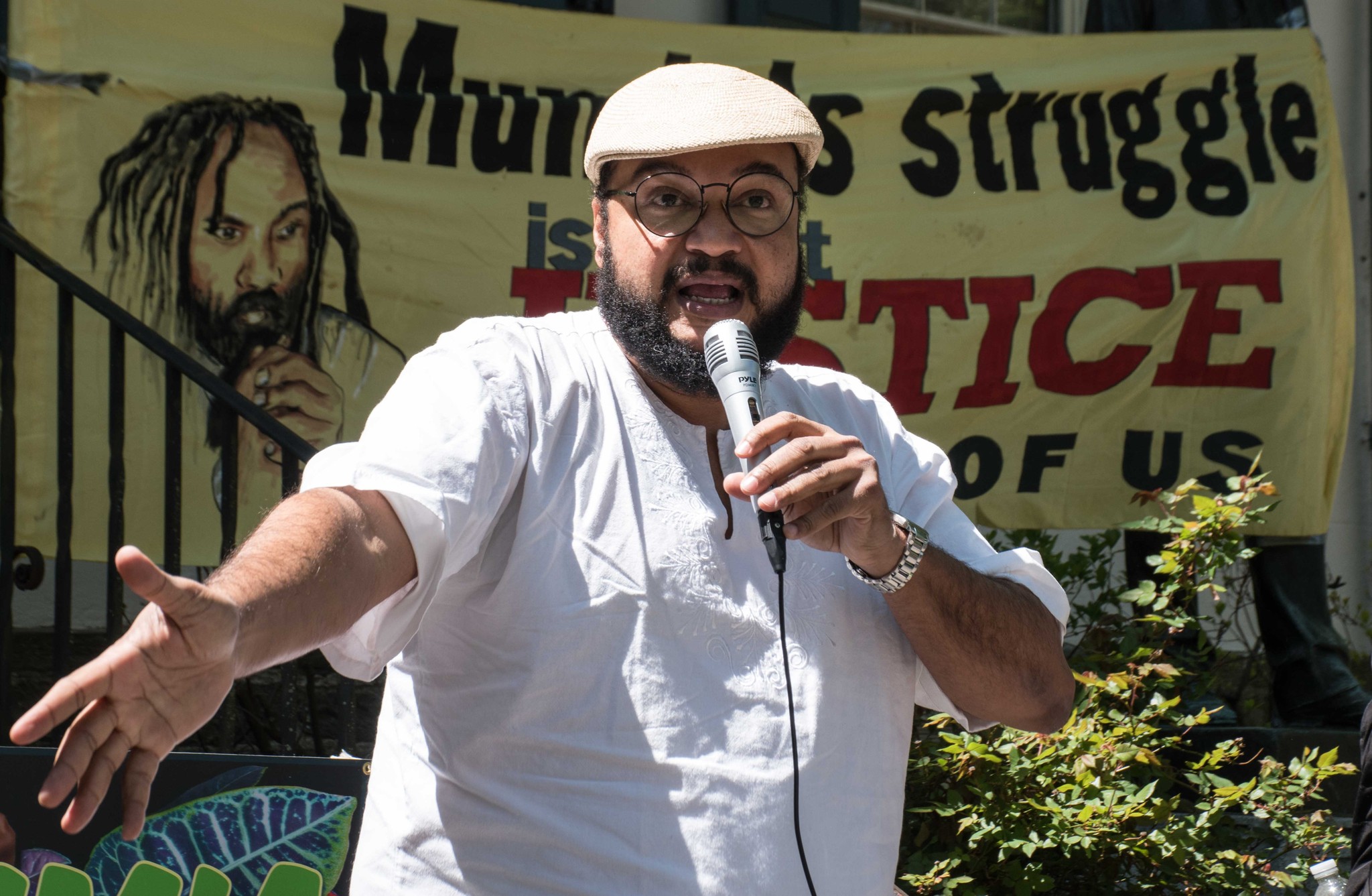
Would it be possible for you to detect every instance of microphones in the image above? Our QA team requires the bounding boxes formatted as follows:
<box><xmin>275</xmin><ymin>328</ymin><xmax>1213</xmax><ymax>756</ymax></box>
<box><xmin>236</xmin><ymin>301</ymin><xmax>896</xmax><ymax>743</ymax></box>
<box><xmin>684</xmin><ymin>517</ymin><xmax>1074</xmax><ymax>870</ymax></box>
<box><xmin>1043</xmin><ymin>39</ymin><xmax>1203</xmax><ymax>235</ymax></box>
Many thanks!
<box><xmin>703</xmin><ymin>317</ymin><xmax>787</xmax><ymax>575</ymax></box>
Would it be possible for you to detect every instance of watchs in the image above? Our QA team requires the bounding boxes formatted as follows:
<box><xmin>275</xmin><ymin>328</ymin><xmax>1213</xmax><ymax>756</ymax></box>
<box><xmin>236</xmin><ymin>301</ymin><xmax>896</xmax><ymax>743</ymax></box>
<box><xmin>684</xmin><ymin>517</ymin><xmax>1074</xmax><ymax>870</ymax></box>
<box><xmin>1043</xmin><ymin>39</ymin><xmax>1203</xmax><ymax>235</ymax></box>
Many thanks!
<box><xmin>844</xmin><ymin>509</ymin><xmax>930</xmax><ymax>594</ymax></box>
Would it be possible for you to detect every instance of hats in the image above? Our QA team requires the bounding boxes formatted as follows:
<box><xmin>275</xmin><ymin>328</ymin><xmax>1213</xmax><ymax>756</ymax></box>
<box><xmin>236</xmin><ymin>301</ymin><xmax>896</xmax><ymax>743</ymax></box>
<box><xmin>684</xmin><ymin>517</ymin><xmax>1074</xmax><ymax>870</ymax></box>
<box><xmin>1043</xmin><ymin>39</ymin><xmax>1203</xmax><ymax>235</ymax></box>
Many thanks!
<box><xmin>584</xmin><ymin>62</ymin><xmax>825</xmax><ymax>194</ymax></box>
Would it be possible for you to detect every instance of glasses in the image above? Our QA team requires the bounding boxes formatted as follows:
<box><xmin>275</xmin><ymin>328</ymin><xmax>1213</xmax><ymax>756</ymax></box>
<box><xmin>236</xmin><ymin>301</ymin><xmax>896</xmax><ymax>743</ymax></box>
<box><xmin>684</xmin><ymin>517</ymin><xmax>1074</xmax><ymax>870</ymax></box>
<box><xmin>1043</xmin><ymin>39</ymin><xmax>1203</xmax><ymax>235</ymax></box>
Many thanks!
<box><xmin>604</xmin><ymin>171</ymin><xmax>800</xmax><ymax>238</ymax></box>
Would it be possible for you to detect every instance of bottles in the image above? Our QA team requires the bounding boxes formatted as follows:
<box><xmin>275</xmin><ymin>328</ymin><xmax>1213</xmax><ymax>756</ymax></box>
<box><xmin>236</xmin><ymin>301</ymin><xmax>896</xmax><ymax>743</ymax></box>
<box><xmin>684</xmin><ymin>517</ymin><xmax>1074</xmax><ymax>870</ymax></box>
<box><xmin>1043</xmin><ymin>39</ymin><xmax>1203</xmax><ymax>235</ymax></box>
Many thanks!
<box><xmin>1309</xmin><ymin>859</ymin><xmax>1349</xmax><ymax>896</ymax></box>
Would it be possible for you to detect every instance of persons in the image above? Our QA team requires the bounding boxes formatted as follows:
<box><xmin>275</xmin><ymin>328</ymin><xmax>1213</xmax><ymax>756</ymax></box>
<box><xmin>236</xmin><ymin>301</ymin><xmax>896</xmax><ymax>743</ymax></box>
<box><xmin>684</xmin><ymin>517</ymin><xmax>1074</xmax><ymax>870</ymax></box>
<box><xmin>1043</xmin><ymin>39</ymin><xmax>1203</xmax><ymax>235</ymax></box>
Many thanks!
<box><xmin>5</xmin><ymin>63</ymin><xmax>1076</xmax><ymax>896</ymax></box>
